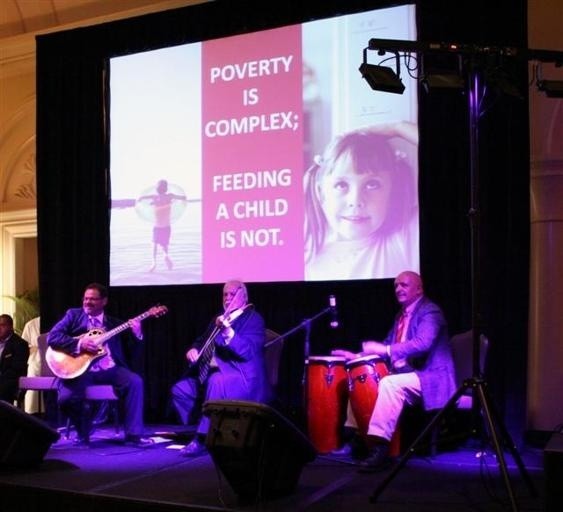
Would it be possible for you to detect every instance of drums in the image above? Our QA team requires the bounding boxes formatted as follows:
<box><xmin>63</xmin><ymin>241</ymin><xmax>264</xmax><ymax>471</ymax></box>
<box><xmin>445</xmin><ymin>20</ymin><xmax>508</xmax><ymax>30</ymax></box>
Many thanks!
<box><xmin>346</xmin><ymin>354</ymin><xmax>403</xmax><ymax>458</ymax></box>
<box><xmin>307</xmin><ymin>356</ymin><xmax>352</xmax><ymax>454</ymax></box>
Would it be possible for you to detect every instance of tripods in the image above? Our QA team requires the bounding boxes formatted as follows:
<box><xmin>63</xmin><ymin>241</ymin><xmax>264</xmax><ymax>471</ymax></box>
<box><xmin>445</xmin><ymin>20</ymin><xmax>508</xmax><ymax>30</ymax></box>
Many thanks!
<box><xmin>368</xmin><ymin>73</ymin><xmax>538</xmax><ymax>512</ymax></box>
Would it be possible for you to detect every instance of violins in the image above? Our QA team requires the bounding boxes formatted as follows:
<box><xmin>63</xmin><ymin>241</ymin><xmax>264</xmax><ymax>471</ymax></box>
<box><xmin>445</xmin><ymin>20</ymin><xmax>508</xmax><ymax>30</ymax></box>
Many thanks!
<box><xmin>216</xmin><ymin>308</ymin><xmax>243</xmax><ymax>331</ymax></box>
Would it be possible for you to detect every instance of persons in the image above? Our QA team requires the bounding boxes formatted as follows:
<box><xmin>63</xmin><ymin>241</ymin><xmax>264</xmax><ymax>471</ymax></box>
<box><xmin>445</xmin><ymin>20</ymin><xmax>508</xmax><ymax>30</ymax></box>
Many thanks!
<box><xmin>170</xmin><ymin>277</ymin><xmax>268</xmax><ymax>458</ymax></box>
<box><xmin>45</xmin><ymin>282</ymin><xmax>154</xmax><ymax>448</ymax></box>
<box><xmin>300</xmin><ymin>120</ymin><xmax>419</xmax><ymax>280</ymax></box>
<box><xmin>135</xmin><ymin>180</ymin><xmax>189</xmax><ymax>273</ymax></box>
<box><xmin>325</xmin><ymin>271</ymin><xmax>459</xmax><ymax>473</ymax></box>
<box><xmin>0</xmin><ymin>311</ymin><xmax>30</xmax><ymax>405</ymax></box>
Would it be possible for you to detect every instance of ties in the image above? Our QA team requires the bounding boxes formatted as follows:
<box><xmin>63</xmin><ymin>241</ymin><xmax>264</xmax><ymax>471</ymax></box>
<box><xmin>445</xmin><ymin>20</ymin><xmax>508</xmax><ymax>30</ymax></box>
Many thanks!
<box><xmin>199</xmin><ymin>317</ymin><xmax>231</xmax><ymax>384</ymax></box>
<box><xmin>395</xmin><ymin>312</ymin><xmax>405</xmax><ymax>343</ymax></box>
<box><xmin>90</xmin><ymin>317</ymin><xmax>96</xmax><ymax>328</ymax></box>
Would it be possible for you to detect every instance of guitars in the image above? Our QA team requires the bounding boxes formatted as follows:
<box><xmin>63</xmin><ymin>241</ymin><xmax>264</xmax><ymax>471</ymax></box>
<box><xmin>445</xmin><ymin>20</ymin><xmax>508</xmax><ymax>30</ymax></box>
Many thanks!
<box><xmin>46</xmin><ymin>305</ymin><xmax>169</xmax><ymax>380</ymax></box>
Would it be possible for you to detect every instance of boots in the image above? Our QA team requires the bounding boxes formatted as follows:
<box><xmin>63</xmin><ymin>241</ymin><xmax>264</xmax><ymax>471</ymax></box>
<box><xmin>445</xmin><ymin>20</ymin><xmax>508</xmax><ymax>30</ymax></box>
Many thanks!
<box><xmin>360</xmin><ymin>444</ymin><xmax>391</xmax><ymax>469</ymax></box>
<box><xmin>329</xmin><ymin>435</ymin><xmax>364</xmax><ymax>457</ymax></box>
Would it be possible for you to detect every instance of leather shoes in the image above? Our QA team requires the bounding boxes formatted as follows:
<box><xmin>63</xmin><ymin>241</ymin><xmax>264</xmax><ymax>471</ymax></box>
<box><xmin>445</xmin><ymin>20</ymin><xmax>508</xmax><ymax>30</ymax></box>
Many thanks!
<box><xmin>125</xmin><ymin>434</ymin><xmax>154</xmax><ymax>447</ymax></box>
<box><xmin>74</xmin><ymin>434</ymin><xmax>89</xmax><ymax>446</ymax></box>
<box><xmin>180</xmin><ymin>440</ymin><xmax>209</xmax><ymax>457</ymax></box>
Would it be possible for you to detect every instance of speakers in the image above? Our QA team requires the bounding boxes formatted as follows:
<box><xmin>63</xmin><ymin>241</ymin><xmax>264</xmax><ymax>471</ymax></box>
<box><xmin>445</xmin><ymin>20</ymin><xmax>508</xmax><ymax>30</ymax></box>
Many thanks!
<box><xmin>0</xmin><ymin>398</ymin><xmax>61</xmax><ymax>475</ymax></box>
<box><xmin>200</xmin><ymin>399</ymin><xmax>318</xmax><ymax>501</ymax></box>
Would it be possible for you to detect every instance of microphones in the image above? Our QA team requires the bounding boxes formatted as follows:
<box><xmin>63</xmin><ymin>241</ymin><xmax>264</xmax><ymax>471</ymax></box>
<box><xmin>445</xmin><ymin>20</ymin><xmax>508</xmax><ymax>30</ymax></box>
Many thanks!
<box><xmin>329</xmin><ymin>294</ymin><xmax>339</xmax><ymax>328</ymax></box>
